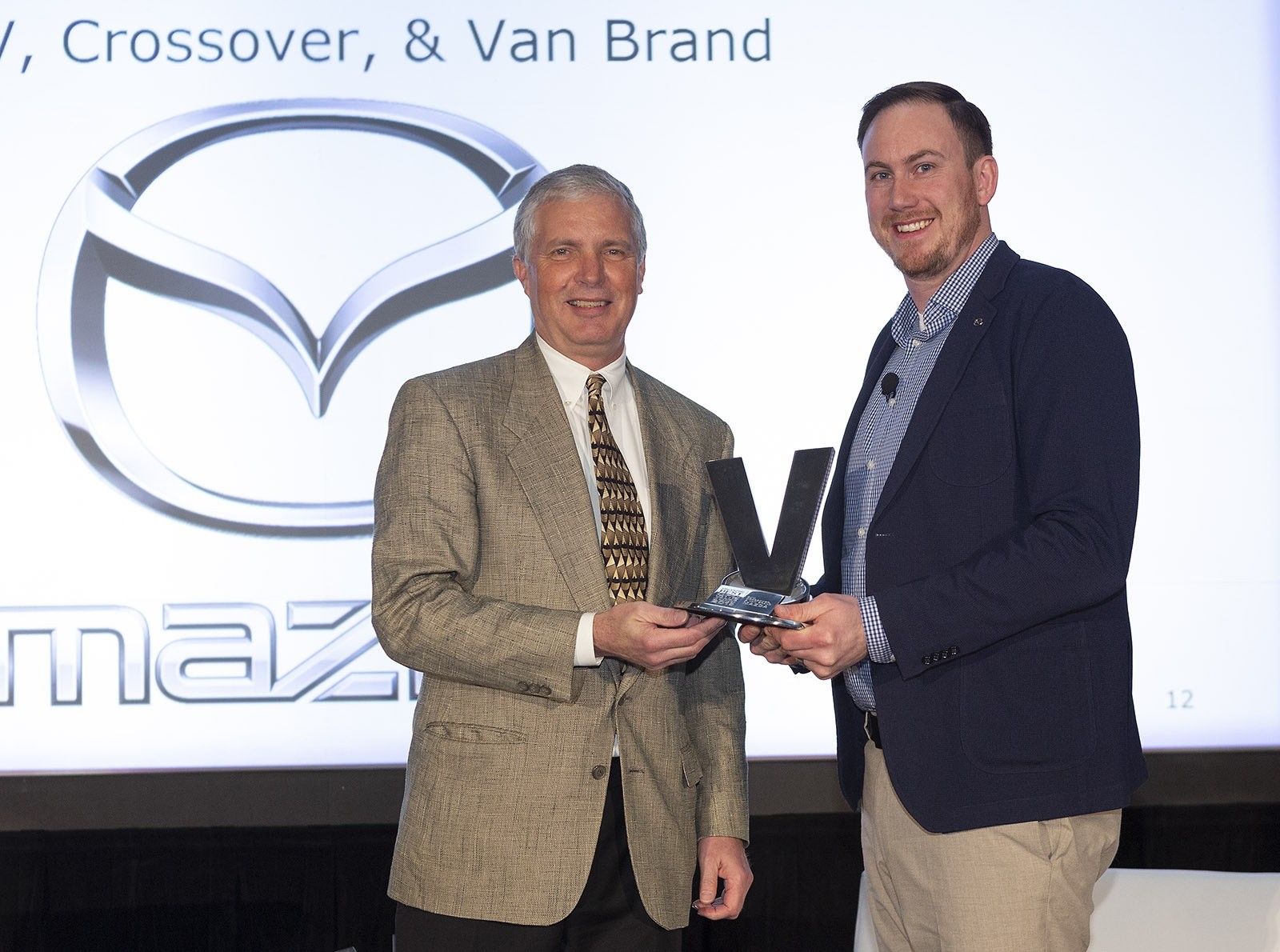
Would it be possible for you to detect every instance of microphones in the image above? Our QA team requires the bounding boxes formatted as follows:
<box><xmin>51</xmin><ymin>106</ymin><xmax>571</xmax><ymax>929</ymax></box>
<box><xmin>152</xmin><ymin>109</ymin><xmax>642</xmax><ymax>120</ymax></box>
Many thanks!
<box><xmin>880</xmin><ymin>372</ymin><xmax>900</xmax><ymax>402</ymax></box>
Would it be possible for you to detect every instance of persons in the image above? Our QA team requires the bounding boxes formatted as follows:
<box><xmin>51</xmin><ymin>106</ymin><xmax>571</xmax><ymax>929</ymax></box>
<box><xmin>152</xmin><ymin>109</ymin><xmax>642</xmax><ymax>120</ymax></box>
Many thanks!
<box><xmin>739</xmin><ymin>80</ymin><xmax>1142</xmax><ymax>952</ymax></box>
<box><xmin>370</xmin><ymin>165</ymin><xmax>754</xmax><ymax>952</ymax></box>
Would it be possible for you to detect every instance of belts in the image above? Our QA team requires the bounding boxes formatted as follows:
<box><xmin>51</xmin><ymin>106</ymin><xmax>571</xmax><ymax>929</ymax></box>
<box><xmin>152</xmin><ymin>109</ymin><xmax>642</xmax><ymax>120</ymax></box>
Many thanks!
<box><xmin>866</xmin><ymin>712</ymin><xmax>883</xmax><ymax>749</ymax></box>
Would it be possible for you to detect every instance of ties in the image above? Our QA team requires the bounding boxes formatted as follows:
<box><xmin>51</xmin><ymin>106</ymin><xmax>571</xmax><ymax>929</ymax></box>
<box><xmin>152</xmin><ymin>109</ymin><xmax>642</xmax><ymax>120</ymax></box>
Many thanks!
<box><xmin>586</xmin><ymin>373</ymin><xmax>649</xmax><ymax>685</ymax></box>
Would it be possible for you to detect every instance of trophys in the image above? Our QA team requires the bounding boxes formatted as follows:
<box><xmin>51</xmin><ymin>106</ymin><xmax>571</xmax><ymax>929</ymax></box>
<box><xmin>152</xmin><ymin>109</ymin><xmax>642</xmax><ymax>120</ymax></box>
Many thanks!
<box><xmin>673</xmin><ymin>447</ymin><xmax>836</xmax><ymax>629</ymax></box>
